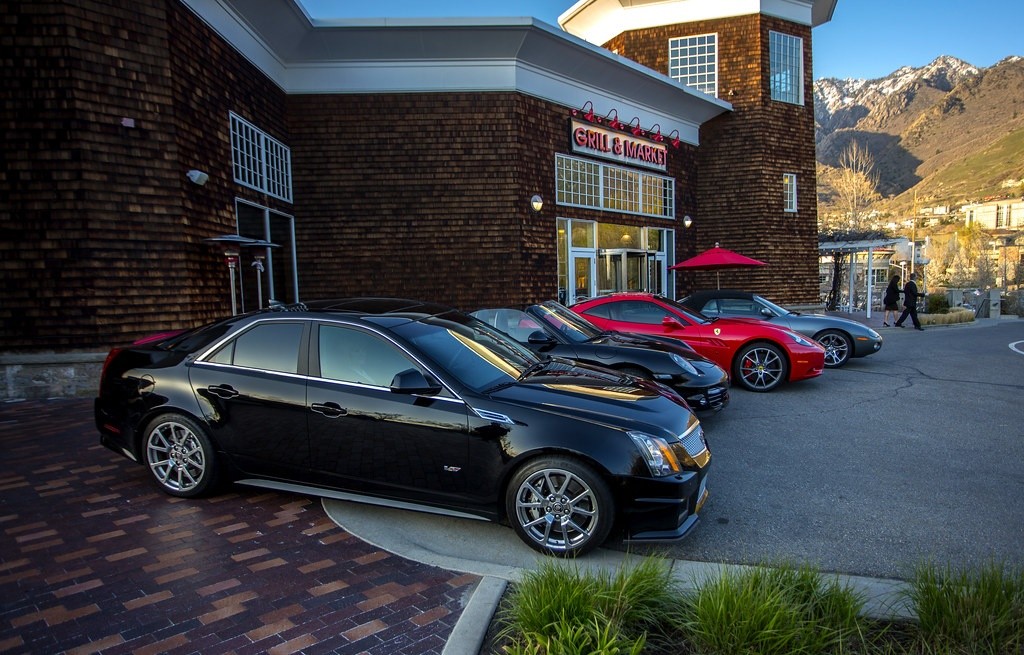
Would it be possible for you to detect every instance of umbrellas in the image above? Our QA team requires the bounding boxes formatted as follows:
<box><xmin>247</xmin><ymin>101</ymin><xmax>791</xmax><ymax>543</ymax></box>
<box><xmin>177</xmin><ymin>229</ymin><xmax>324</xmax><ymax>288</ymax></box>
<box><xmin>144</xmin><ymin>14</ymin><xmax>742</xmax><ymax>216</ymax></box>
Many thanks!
<box><xmin>666</xmin><ymin>242</ymin><xmax>769</xmax><ymax>291</ymax></box>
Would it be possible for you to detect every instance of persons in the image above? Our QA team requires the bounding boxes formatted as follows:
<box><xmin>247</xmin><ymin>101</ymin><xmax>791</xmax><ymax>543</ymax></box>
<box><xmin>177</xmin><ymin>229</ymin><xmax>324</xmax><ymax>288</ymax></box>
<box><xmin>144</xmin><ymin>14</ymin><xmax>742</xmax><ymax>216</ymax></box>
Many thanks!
<box><xmin>895</xmin><ymin>272</ymin><xmax>926</xmax><ymax>331</ymax></box>
<box><xmin>883</xmin><ymin>275</ymin><xmax>904</xmax><ymax>327</ymax></box>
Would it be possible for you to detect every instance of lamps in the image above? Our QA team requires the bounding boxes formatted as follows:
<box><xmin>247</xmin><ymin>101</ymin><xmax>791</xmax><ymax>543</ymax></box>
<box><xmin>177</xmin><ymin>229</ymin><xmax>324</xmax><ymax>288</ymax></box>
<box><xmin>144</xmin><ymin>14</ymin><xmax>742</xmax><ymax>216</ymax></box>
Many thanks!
<box><xmin>683</xmin><ymin>215</ymin><xmax>693</xmax><ymax>228</ymax></box>
<box><xmin>641</xmin><ymin>123</ymin><xmax>662</xmax><ymax>142</ymax></box>
<box><xmin>186</xmin><ymin>170</ymin><xmax>208</xmax><ymax>186</ymax></box>
<box><xmin>664</xmin><ymin>129</ymin><xmax>680</xmax><ymax>149</ymax></box>
<box><xmin>571</xmin><ymin>101</ymin><xmax>594</xmax><ymax>123</ymax></box>
<box><xmin>620</xmin><ymin>116</ymin><xmax>640</xmax><ymax>137</ymax></box>
<box><xmin>531</xmin><ymin>195</ymin><xmax>543</xmax><ymax>212</ymax></box>
<box><xmin>597</xmin><ymin>109</ymin><xmax>619</xmax><ymax>131</ymax></box>
<box><xmin>620</xmin><ymin>234</ymin><xmax>635</xmax><ymax>245</ymax></box>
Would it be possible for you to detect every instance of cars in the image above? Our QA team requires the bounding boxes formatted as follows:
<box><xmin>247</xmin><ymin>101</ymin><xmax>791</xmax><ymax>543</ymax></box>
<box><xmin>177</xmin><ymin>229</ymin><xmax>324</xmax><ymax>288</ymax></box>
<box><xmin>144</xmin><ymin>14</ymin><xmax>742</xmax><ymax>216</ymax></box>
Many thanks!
<box><xmin>93</xmin><ymin>297</ymin><xmax>714</xmax><ymax>560</ymax></box>
<box><xmin>961</xmin><ymin>303</ymin><xmax>975</xmax><ymax>313</ymax></box>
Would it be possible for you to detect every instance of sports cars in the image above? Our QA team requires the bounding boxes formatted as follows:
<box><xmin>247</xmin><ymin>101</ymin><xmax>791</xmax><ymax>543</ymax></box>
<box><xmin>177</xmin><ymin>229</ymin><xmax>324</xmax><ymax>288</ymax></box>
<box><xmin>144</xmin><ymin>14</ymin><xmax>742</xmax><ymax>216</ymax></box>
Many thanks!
<box><xmin>518</xmin><ymin>290</ymin><xmax>826</xmax><ymax>392</ymax></box>
<box><xmin>464</xmin><ymin>299</ymin><xmax>731</xmax><ymax>419</ymax></box>
<box><xmin>662</xmin><ymin>290</ymin><xmax>882</xmax><ymax>368</ymax></box>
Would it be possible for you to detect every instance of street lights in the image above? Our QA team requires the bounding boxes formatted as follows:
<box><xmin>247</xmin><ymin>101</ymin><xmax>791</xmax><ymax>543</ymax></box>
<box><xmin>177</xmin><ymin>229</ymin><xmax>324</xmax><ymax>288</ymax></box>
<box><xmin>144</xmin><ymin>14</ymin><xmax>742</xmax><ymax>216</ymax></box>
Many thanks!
<box><xmin>921</xmin><ymin>244</ymin><xmax>928</xmax><ymax>313</ymax></box>
<box><xmin>907</xmin><ymin>242</ymin><xmax>915</xmax><ymax>275</ymax></box>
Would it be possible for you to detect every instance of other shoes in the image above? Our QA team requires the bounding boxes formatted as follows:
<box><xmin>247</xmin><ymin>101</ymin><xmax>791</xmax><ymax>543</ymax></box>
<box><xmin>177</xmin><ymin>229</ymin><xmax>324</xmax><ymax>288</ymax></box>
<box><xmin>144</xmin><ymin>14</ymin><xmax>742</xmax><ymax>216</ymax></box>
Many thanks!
<box><xmin>895</xmin><ymin>324</ymin><xmax>906</xmax><ymax>328</ymax></box>
<box><xmin>914</xmin><ymin>326</ymin><xmax>925</xmax><ymax>331</ymax></box>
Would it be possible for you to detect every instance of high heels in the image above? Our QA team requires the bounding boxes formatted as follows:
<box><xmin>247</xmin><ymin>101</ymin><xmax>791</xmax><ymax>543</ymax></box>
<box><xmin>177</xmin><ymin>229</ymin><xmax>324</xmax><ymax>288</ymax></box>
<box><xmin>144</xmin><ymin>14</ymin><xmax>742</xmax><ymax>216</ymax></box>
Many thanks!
<box><xmin>884</xmin><ymin>322</ymin><xmax>890</xmax><ymax>327</ymax></box>
<box><xmin>894</xmin><ymin>322</ymin><xmax>897</xmax><ymax>324</ymax></box>
